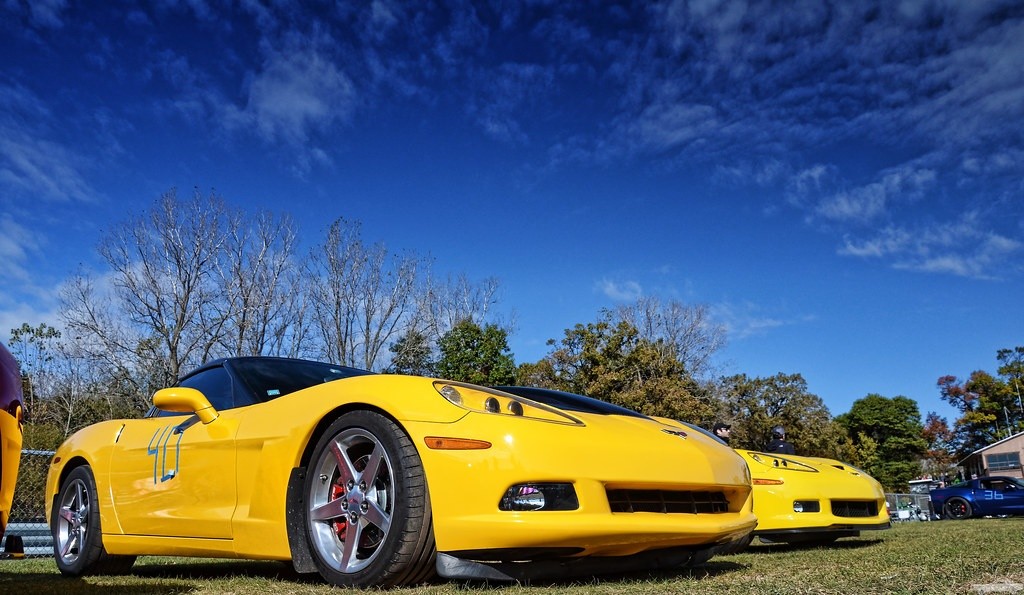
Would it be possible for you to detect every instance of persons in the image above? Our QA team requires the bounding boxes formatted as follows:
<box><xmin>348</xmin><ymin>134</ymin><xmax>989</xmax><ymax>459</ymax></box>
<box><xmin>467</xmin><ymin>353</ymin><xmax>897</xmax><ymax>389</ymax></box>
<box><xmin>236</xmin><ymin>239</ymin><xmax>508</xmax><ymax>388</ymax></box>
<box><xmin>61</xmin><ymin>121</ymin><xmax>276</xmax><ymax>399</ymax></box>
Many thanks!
<box><xmin>915</xmin><ymin>469</ymin><xmax>987</xmax><ymax>486</ymax></box>
<box><xmin>766</xmin><ymin>426</ymin><xmax>793</xmax><ymax>454</ymax></box>
<box><xmin>713</xmin><ymin>423</ymin><xmax>732</xmax><ymax>447</ymax></box>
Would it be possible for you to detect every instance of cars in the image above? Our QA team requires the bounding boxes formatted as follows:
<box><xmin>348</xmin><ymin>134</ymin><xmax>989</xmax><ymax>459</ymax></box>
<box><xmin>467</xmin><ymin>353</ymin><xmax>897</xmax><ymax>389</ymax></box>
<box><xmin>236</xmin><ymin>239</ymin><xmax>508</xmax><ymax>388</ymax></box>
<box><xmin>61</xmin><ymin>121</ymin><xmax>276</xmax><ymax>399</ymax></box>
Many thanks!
<box><xmin>930</xmin><ymin>475</ymin><xmax>1024</xmax><ymax>519</ymax></box>
<box><xmin>719</xmin><ymin>448</ymin><xmax>892</xmax><ymax>556</ymax></box>
<box><xmin>46</xmin><ymin>355</ymin><xmax>757</xmax><ymax>589</ymax></box>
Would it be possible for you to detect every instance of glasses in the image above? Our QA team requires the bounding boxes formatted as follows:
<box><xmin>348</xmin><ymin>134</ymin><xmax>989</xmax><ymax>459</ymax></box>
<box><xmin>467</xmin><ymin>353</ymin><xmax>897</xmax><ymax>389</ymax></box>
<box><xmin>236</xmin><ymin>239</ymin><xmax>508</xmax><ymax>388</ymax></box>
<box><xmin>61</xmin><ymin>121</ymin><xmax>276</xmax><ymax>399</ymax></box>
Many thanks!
<box><xmin>718</xmin><ymin>428</ymin><xmax>727</xmax><ymax>431</ymax></box>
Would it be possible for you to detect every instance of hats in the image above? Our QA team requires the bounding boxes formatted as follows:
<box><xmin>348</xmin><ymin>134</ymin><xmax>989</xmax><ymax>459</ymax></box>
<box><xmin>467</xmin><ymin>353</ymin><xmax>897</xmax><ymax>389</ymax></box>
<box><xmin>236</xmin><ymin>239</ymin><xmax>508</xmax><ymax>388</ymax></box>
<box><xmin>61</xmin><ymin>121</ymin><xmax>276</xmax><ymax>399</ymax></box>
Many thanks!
<box><xmin>773</xmin><ymin>426</ymin><xmax>788</xmax><ymax>437</ymax></box>
<box><xmin>713</xmin><ymin>423</ymin><xmax>731</xmax><ymax>433</ymax></box>
